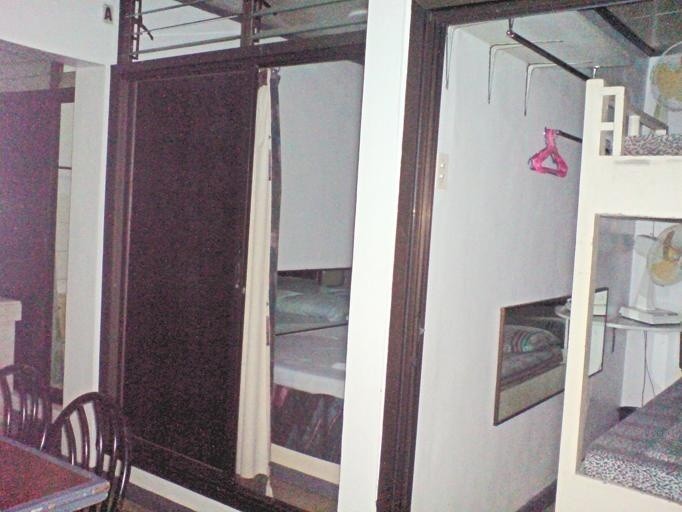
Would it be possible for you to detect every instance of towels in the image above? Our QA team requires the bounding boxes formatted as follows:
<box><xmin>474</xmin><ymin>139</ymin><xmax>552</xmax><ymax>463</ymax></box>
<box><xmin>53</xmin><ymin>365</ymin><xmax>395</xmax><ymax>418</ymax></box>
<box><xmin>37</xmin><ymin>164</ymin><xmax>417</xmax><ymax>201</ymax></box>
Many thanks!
<box><xmin>501</xmin><ymin>324</ymin><xmax>562</xmax><ymax>355</ymax></box>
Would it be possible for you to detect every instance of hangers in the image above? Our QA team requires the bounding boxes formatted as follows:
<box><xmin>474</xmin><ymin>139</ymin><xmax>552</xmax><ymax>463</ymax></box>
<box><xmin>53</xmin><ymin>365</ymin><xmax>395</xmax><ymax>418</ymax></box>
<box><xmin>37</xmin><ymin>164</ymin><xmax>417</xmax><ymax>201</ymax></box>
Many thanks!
<box><xmin>529</xmin><ymin>127</ymin><xmax>568</xmax><ymax>176</ymax></box>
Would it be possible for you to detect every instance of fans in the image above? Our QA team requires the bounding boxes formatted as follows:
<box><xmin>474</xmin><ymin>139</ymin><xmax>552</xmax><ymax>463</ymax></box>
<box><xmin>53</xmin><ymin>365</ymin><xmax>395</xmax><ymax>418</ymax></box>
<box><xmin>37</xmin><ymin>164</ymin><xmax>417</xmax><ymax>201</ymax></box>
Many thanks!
<box><xmin>652</xmin><ymin>40</ymin><xmax>682</xmax><ymax>118</ymax></box>
<box><xmin>619</xmin><ymin>223</ymin><xmax>682</xmax><ymax>325</ymax></box>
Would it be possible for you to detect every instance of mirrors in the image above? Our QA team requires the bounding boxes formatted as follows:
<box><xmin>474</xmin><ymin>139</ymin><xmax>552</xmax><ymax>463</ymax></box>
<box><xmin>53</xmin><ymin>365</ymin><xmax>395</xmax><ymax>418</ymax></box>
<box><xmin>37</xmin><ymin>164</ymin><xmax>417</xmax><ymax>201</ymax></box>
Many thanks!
<box><xmin>493</xmin><ymin>286</ymin><xmax>610</xmax><ymax>427</ymax></box>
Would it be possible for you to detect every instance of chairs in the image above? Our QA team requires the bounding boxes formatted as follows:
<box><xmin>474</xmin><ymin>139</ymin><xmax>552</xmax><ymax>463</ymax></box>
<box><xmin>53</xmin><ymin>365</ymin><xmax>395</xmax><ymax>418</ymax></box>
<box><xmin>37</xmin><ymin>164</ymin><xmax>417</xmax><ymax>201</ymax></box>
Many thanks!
<box><xmin>0</xmin><ymin>362</ymin><xmax>52</xmax><ymax>451</ymax></box>
<box><xmin>40</xmin><ymin>391</ymin><xmax>131</xmax><ymax>511</ymax></box>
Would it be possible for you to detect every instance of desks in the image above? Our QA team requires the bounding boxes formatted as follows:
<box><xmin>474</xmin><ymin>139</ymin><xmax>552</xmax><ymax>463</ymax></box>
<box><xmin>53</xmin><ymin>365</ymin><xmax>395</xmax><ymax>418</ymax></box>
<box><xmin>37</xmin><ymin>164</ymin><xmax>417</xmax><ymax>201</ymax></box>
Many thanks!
<box><xmin>0</xmin><ymin>435</ymin><xmax>112</xmax><ymax>512</ymax></box>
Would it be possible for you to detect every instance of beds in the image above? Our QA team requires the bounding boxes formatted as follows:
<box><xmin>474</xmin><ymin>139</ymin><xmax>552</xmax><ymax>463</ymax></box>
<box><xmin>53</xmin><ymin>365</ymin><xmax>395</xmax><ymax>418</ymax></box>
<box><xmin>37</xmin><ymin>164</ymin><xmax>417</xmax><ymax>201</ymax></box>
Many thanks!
<box><xmin>272</xmin><ymin>276</ymin><xmax>350</xmax><ymax>502</ymax></box>
<box><xmin>555</xmin><ymin>77</ymin><xmax>682</xmax><ymax>510</ymax></box>
